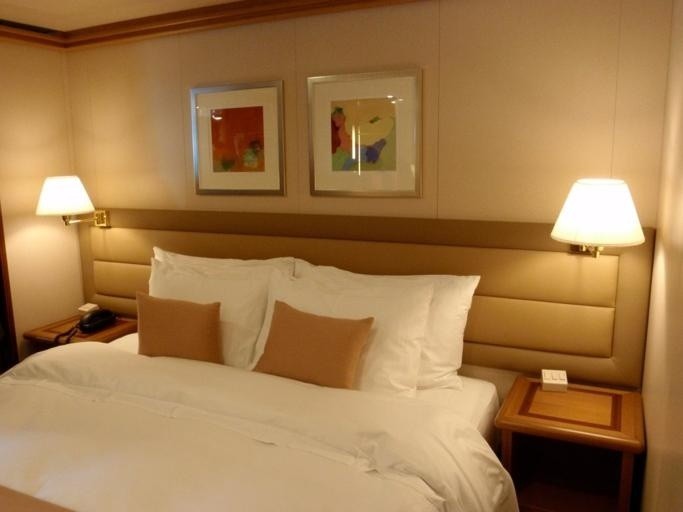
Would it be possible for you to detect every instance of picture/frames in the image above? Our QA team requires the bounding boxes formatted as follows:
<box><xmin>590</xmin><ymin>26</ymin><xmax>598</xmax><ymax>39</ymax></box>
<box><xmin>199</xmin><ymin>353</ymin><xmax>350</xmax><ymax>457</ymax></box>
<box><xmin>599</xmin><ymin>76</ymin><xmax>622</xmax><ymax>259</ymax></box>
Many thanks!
<box><xmin>187</xmin><ymin>78</ymin><xmax>287</xmax><ymax>196</ymax></box>
<box><xmin>305</xmin><ymin>68</ymin><xmax>423</xmax><ymax>201</ymax></box>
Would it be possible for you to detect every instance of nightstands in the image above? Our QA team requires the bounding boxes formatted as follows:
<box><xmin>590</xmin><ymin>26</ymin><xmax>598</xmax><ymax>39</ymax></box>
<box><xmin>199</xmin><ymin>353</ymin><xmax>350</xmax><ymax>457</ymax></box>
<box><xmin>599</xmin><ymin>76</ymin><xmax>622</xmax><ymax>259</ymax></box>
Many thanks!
<box><xmin>491</xmin><ymin>374</ymin><xmax>644</xmax><ymax>511</ymax></box>
<box><xmin>22</xmin><ymin>310</ymin><xmax>137</xmax><ymax>356</ymax></box>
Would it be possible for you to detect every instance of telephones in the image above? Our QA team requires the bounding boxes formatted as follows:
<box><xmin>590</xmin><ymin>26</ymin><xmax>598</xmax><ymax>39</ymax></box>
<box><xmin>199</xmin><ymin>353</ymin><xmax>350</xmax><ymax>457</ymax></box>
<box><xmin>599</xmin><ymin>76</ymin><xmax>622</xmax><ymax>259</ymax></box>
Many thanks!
<box><xmin>76</xmin><ymin>308</ymin><xmax>116</xmax><ymax>334</ymax></box>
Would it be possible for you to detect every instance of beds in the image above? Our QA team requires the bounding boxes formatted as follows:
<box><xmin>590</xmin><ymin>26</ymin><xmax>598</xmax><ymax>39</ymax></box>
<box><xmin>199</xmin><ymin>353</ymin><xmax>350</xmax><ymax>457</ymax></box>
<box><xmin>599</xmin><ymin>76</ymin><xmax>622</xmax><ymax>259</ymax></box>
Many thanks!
<box><xmin>0</xmin><ymin>331</ymin><xmax>520</xmax><ymax>510</ymax></box>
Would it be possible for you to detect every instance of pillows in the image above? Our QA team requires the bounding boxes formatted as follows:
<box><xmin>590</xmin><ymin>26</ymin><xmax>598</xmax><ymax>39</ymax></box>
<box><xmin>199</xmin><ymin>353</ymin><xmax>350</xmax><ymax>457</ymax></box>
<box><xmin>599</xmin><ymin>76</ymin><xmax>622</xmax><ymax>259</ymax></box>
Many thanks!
<box><xmin>151</xmin><ymin>244</ymin><xmax>291</xmax><ymax>277</ymax></box>
<box><xmin>133</xmin><ymin>292</ymin><xmax>225</xmax><ymax>364</ymax></box>
<box><xmin>253</xmin><ymin>267</ymin><xmax>431</xmax><ymax>396</ymax></box>
<box><xmin>148</xmin><ymin>257</ymin><xmax>280</xmax><ymax>368</ymax></box>
<box><xmin>250</xmin><ymin>299</ymin><xmax>374</xmax><ymax>391</ymax></box>
<box><xmin>293</xmin><ymin>254</ymin><xmax>483</xmax><ymax>390</ymax></box>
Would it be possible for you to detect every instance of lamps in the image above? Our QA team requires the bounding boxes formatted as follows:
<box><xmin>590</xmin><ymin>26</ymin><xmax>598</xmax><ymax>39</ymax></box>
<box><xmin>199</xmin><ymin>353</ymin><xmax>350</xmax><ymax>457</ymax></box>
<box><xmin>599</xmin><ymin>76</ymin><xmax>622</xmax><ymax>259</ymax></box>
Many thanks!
<box><xmin>549</xmin><ymin>177</ymin><xmax>646</xmax><ymax>259</ymax></box>
<box><xmin>35</xmin><ymin>174</ymin><xmax>108</xmax><ymax>228</ymax></box>
